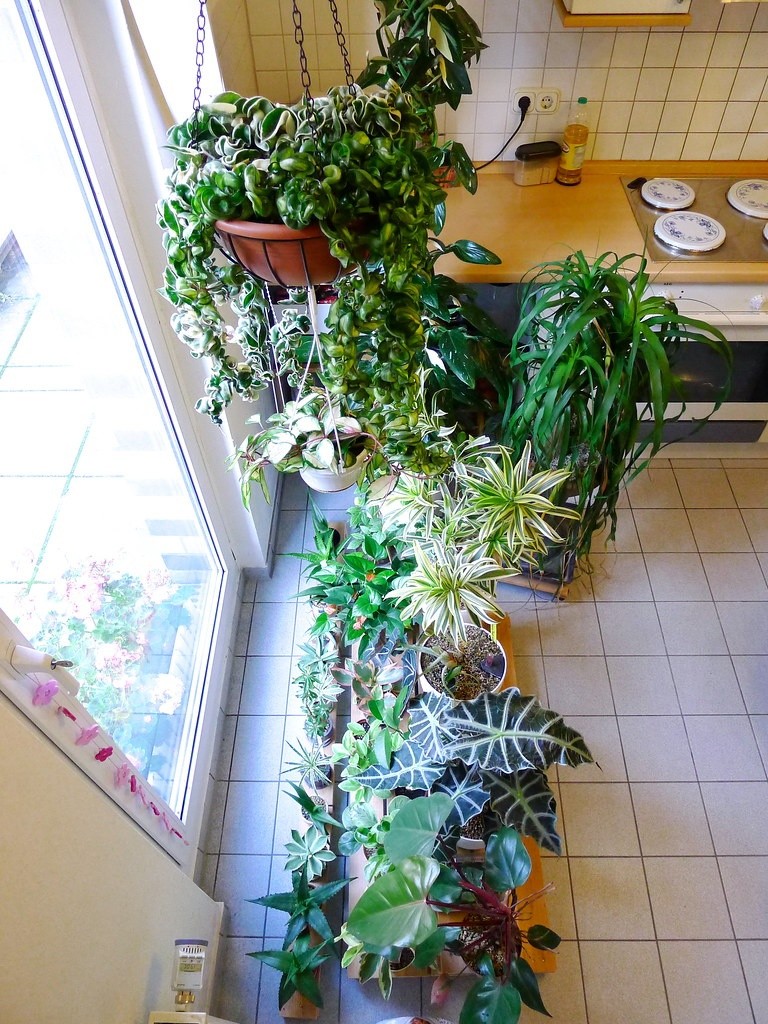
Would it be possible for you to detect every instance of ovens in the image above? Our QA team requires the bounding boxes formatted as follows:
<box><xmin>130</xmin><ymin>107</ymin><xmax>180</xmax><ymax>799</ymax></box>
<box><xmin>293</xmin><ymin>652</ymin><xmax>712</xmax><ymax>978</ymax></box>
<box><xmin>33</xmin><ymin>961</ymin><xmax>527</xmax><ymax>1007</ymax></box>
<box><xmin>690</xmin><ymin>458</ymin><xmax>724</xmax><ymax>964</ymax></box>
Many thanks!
<box><xmin>634</xmin><ymin>285</ymin><xmax>768</xmax><ymax>443</ymax></box>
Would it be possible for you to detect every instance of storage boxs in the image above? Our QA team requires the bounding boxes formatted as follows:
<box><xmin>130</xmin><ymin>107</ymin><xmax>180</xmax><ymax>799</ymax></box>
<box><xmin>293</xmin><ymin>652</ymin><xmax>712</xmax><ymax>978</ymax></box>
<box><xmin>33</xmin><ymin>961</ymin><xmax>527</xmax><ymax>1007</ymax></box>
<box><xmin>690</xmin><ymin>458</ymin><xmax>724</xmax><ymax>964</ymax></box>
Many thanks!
<box><xmin>514</xmin><ymin>141</ymin><xmax>561</xmax><ymax>185</ymax></box>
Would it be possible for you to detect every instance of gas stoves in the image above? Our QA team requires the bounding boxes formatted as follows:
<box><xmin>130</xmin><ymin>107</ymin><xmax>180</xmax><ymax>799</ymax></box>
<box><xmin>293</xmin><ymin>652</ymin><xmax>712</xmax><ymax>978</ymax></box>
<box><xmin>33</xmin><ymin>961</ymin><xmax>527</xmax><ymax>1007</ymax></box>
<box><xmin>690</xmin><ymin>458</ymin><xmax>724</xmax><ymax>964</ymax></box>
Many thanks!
<box><xmin>619</xmin><ymin>175</ymin><xmax>768</xmax><ymax>262</ymax></box>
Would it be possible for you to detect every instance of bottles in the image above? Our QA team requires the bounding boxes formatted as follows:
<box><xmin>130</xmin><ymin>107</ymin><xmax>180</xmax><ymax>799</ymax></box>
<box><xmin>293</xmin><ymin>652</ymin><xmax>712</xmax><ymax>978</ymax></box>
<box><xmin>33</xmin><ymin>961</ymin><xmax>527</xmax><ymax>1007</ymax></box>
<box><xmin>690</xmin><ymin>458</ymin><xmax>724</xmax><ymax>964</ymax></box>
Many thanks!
<box><xmin>556</xmin><ymin>97</ymin><xmax>591</xmax><ymax>186</ymax></box>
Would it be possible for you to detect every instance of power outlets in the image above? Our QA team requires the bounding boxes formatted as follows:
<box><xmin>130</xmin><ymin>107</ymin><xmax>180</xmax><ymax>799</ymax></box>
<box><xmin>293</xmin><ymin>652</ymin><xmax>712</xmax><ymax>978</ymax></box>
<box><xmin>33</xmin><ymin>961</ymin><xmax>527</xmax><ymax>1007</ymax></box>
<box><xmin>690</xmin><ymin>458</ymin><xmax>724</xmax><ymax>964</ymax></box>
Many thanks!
<box><xmin>512</xmin><ymin>88</ymin><xmax>562</xmax><ymax>115</ymax></box>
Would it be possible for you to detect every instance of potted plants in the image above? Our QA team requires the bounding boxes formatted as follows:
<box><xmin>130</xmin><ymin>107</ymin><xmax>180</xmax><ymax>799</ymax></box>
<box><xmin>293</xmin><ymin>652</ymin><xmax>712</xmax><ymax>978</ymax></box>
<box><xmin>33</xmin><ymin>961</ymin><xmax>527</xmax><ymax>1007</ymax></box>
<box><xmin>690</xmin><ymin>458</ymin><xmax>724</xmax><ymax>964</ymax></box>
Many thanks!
<box><xmin>155</xmin><ymin>0</ymin><xmax>735</xmax><ymax>603</ymax></box>
<box><xmin>242</xmin><ymin>441</ymin><xmax>602</xmax><ymax>1024</ymax></box>
